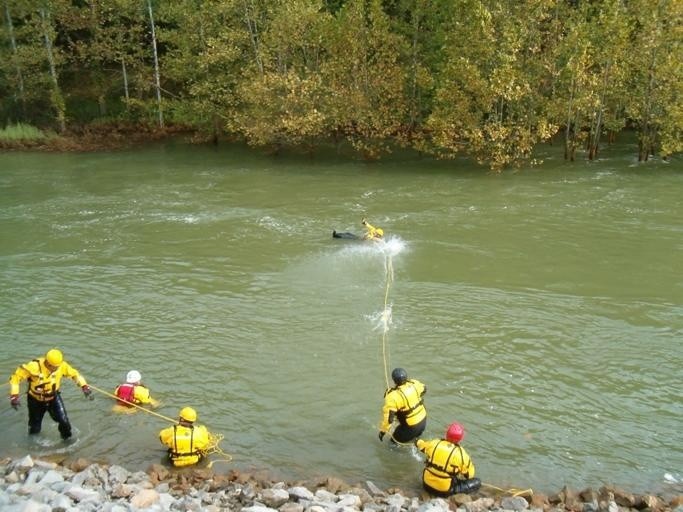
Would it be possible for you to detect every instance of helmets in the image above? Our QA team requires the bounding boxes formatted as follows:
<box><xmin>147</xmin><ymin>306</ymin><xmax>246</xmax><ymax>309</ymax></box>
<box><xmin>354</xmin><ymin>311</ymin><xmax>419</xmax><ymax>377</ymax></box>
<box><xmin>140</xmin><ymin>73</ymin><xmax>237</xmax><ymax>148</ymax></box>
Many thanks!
<box><xmin>47</xmin><ymin>350</ymin><xmax>62</xmax><ymax>367</ymax></box>
<box><xmin>179</xmin><ymin>407</ymin><xmax>195</xmax><ymax>422</ymax></box>
<box><xmin>374</xmin><ymin>229</ymin><xmax>383</xmax><ymax>236</ymax></box>
<box><xmin>446</xmin><ymin>424</ymin><xmax>463</xmax><ymax>443</ymax></box>
<box><xmin>391</xmin><ymin>368</ymin><xmax>407</xmax><ymax>383</ymax></box>
<box><xmin>126</xmin><ymin>370</ymin><xmax>141</xmax><ymax>383</ymax></box>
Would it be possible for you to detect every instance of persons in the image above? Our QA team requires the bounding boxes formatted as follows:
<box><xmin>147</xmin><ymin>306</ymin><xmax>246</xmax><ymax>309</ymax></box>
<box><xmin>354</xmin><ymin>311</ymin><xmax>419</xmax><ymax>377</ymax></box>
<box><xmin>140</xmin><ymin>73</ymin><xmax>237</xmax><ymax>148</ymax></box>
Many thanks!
<box><xmin>8</xmin><ymin>349</ymin><xmax>93</xmax><ymax>440</ymax></box>
<box><xmin>157</xmin><ymin>406</ymin><xmax>209</xmax><ymax>466</ymax></box>
<box><xmin>111</xmin><ymin>369</ymin><xmax>159</xmax><ymax>416</ymax></box>
<box><xmin>377</xmin><ymin>367</ymin><xmax>427</xmax><ymax>446</ymax></box>
<box><xmin>410</xmin><ymin>423</ymin><xmax>481</xmax><ymax>497</ymax></box>
<box><xmin>331</xmin><ymin>217</ymin><xmax>384</xmax><ymax>242</ymax></box>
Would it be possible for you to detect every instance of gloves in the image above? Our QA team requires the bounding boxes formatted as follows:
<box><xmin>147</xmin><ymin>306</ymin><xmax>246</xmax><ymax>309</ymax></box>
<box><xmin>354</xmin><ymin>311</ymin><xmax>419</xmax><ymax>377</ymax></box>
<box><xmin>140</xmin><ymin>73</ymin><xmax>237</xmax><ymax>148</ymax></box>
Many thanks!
<box><xmin>83</xmin><ymin>385</ymin><xmax>94</xmax><ymax>400</ymax></box>
<box><xmin>11</xmin><ymin>395</ymin><xmax>20</xmax><ymax>410</ymax></box>
<box><xmin>378</xmin><ymin>432</ymin><xmax>384</xmax><ymax>441</ymax></box>
